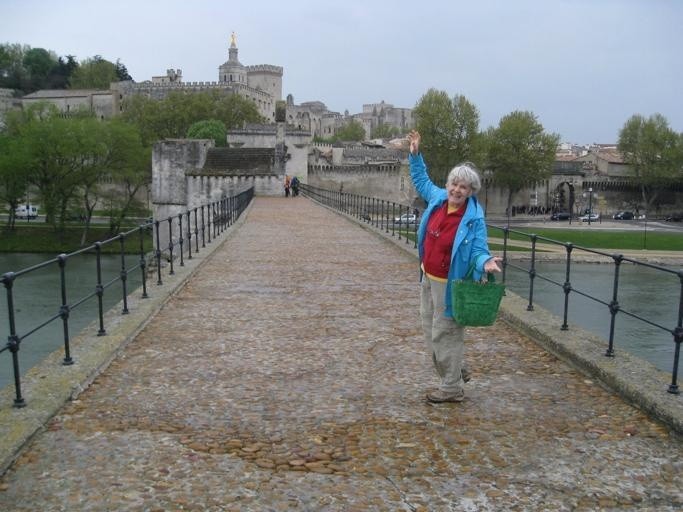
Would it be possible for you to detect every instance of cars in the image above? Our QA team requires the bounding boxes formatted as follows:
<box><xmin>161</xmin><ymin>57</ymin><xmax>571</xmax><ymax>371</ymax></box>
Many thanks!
<box><xmin>552</xmin><ymin>211</ymin><xmax>574</xmax><ymax>221</ymax></box>
<box><xmin>576</xmin><ymin>213</ymin><xmax>599</xmax><ymax>223</ymax></box>
<box><xmin>359</xmin><ymin>214</ymin><xmax>370</xmax><ymax>220</ymax></box>
<box><xmin>664</xmin><ymin>213</ymin><xmax>682</xmax><ymax>223</ymax></box>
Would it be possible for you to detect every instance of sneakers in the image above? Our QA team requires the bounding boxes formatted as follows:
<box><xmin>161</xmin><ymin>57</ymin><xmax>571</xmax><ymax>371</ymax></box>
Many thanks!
<box><xmin>460</xmin><ymin>368</ymin><xmax>471</xmax><ymax>383</ymax></box>
<box><xmin>425</xmin><ymin>389</ymin><xmax>463</xmax><ymax>403</ymax></box>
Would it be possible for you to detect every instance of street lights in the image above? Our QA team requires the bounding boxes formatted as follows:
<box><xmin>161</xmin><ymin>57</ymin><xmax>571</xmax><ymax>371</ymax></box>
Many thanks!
<box><xmin>587</xmin><ymin>188</ymin><xmax>592</xmax><ymax>224</ymax></box>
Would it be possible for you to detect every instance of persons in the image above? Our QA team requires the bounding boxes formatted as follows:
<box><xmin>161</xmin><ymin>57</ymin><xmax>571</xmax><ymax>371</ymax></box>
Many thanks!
<box><xmin>289</xmin><ymin>177</ymin><xmax>299</xmax><ymax>198</ymax></box>
<box><xmin>283</xmin><ymin>174</ymin><xmax>291</xmax><ymax>197</ymax></box>
<box><xmin>505</xmin><ymin>204</ymin><xmax>555</xmax><ymax>216</ymax></box>
<box><xmin>408</xmin><ymin>130</ymin><xmax>502</xmax><ymax>404</ymax></box>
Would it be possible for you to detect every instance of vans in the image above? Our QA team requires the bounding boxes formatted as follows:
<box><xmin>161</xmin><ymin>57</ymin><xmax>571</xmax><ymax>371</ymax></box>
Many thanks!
<box><xmin>14</xmin><ymin>205</ymin><xmax>37</xmax><ymax>219</ymax></box>
<box><xmin>613</xmin><ymin>211</ymin><xmax>634</xmax><ymax>220</ymax></box>
<box><xmin>395</xmin><ymin>214</ymin><xmax>416</xmax><ymax>224</ymax></box>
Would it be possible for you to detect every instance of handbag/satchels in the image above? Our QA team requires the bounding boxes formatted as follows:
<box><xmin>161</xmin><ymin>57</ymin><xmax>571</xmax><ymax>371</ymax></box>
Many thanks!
<box><xmin>449</xmin><ymin>258</ymin><xmax>506</xmax><ymax>328</ymax></box>
